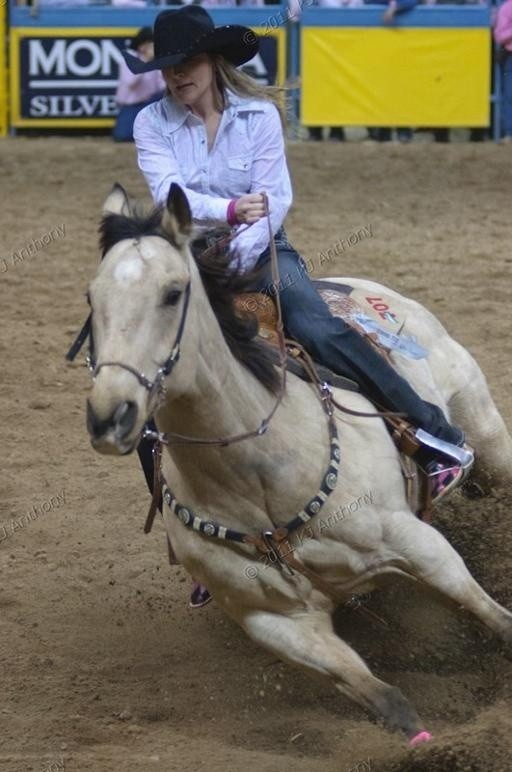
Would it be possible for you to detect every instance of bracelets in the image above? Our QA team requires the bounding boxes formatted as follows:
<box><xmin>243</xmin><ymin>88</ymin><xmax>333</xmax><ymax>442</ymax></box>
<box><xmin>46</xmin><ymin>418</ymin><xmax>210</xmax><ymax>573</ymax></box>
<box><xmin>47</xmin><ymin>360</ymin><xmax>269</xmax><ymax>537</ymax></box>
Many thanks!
<box><xmin>226</xmin><ymin>197</ymin><xmax>241</xmax><ymax>230</ymax></box>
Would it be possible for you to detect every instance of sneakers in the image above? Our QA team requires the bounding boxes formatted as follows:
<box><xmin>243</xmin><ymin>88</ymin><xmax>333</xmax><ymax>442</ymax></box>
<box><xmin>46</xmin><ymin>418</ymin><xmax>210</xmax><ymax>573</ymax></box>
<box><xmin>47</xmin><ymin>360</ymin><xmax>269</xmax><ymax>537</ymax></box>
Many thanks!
<box><xmin>189</xmin><ymin>580</ymin><xmax>212</xmax><ymax>608</ymax></box>
<box><xmin>428</xmin><ymin>442</ymin><xmax>476</xmax><ymax>505</ymax></box>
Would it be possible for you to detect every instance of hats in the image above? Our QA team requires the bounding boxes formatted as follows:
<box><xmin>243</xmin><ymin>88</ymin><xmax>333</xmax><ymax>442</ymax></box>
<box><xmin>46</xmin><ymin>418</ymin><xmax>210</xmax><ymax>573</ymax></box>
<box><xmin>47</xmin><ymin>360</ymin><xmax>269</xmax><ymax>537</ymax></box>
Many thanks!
<box><xmin>129</xmin><ymin>26</ymin><xmax>155</xmax><ymax>50</ymax></box>
<box><xmin>122</xmin><ymin>4</ymin><xmax>260</xmax><ymax>74</ymax></box>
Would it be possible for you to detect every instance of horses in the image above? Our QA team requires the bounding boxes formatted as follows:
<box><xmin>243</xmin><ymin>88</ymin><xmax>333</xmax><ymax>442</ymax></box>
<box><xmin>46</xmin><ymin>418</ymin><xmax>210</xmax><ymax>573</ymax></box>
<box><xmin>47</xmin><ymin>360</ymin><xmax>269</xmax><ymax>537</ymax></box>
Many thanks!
<box><xmin>83</xmin><ymin>179</ymin><xmax>512</xmax><ymax>746</ymax></box>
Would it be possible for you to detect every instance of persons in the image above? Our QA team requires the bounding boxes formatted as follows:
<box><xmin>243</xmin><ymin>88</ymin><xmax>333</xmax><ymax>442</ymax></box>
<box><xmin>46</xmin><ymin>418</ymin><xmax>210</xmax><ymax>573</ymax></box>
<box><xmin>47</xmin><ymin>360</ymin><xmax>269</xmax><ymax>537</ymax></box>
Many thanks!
<box><xmin>115</xmin><ymin>26</ymin><xmax>169</xmax><ymax>144</ymax></box>
<box><xmin>284</xmin><ymin>0</ymin><xmax>512</xmax><ymax>145</ymax></box>
<box><xmin>15</xmin><ymin>0</ymin><xmax>287</xmax><ymax>21</ymax></box>
<box><xmin>133</xmin><ymin>5</ymin><xmax>467</xmax><ymax>610</ymax></box>
